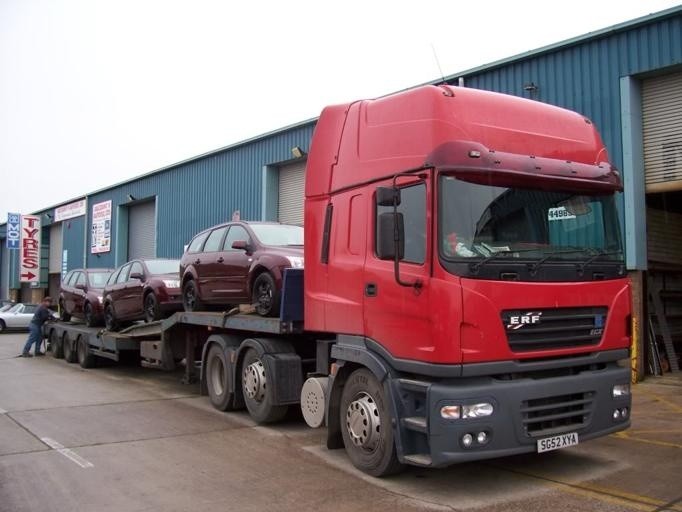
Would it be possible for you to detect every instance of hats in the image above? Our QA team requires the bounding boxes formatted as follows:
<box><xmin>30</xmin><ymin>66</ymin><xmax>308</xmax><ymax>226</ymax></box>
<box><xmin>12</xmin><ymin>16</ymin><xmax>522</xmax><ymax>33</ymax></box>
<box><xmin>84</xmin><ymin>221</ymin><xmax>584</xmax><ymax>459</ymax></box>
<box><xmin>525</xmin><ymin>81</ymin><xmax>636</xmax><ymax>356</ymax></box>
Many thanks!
<box><xmin>57</xmin><ymin>267</ymin><xmax>115</xmax><ymax>327</ymax></box>
<box><xmin>180</xmin><ymin>220</ymin><xmax>304</xmax><ymax>318</ymax></box>
<box><xmin>103</xmin><ymin>257</ymin><xmax>180</xmax><ymax>331</ymax></box>
<box><xmin>0</xmin><ymin>303</ymin><xmax>43</xmax><ymax>333</ymax></box>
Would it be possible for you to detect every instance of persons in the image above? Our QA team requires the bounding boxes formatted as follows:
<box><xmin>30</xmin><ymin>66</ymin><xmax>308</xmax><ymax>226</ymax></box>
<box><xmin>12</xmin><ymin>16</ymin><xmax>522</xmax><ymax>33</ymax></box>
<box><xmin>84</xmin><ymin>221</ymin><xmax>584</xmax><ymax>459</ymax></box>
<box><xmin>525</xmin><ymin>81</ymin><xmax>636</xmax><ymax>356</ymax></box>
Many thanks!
<box><xmin>19</xmin><ymin>295</ymin><xmax>58</xmax><ymax>358</ymax></box>
